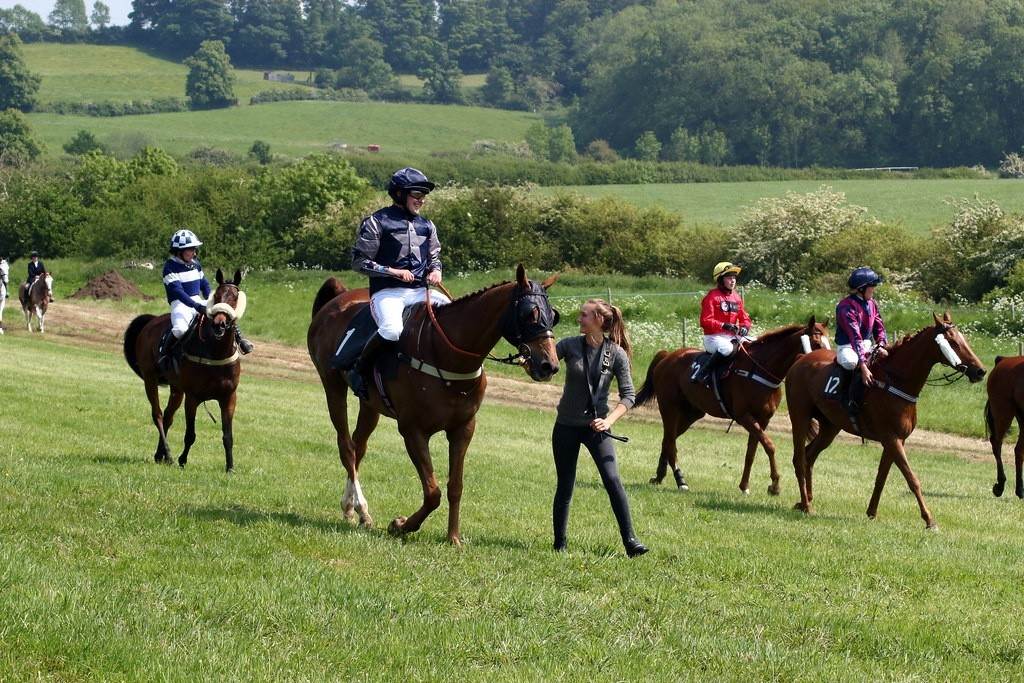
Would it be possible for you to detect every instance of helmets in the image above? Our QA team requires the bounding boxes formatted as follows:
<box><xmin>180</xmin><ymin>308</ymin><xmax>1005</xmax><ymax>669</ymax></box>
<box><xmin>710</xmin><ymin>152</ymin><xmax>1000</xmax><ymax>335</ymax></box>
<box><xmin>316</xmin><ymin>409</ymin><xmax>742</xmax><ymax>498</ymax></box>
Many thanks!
<box><xmin>388</xmin><ymin>167</ymin><xmax>435</xmax><ymax>194</ymax></box>
<box><xmin>712</xmin><ymin>261</ymin><xmax>741</xmax><ymax>283</ymax></box>
<box><xmin>171</xmin><ymin>230</ymin><xmax>203</xmax><ymax>249</ymax></box>
<box><xmin>31</xmin><ymin>251</ymin><xmax>39</xmax><ymax>257</ymax></box>
<box><xmin>848</xmin><ymin>268</ymin><xmax>883</xmax><ymax>289</ymax></box>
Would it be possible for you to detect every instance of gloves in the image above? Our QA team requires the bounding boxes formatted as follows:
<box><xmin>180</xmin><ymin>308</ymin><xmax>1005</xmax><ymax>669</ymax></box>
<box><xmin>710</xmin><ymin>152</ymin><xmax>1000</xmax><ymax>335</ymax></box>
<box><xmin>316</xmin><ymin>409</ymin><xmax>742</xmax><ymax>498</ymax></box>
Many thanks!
<box><xmin>739</xmin><ymin>326</ymin><xmax>748</xmax><ymax>337</ymax></box>
<box><xmin>724</xmin><ymin>324</ymin><xmax>738</xmax><ymax>332</ymax></box>
<box><xmin>198</xmin><ymin>305</ymin><xmax>206</xmax><ymax>315</ymax></box>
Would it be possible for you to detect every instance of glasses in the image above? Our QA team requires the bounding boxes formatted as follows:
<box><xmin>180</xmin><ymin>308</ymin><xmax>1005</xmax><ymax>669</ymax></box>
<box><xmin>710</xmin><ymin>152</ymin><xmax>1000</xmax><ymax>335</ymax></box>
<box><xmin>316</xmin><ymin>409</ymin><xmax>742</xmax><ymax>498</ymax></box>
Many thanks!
<box><xmin>407</xmin><ymin>189</ymin><xmax>426</xmax><ymax>199</ymax></box>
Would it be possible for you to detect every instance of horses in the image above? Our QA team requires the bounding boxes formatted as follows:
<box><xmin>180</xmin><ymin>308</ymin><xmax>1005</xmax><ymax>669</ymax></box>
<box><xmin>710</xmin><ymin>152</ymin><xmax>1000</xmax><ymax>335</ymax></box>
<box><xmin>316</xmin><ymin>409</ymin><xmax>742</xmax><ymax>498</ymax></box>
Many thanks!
<box><xmin>123</xmin><ymin>268</ymin><xmax>240</xmax><ymax>472</ymax></box>
<box><xmin>19</xmin><ymin>269</ymin><xmax>53</xmax><ymax>333</ymax></box>
<box><xmin>785</xmin><ymin>312</ymin><xmax>987</xmax><ymax>533</ymax></box>
<box><xmin>984</xmin><ymin>355</ymin><xmax>1024</xmax><ymax>499</ymax></box>
<box><xmin>8</xmin><ymin>316</ymin><xmax>10</xmax><ymax>317</ymax></box>
<box><xmin>0</xmin><ymin>257</ymin><xmax>9</xmax><ymax>335</ymax></box>
<box><xmin>632</xmin><ymin>315</ymin><xmax>833</xmax><ymax>498</ymax></box>
<box><xmin>306</xmin><ymin>262</ymin><xmax>560</xmax><ymax>546</ymax></box>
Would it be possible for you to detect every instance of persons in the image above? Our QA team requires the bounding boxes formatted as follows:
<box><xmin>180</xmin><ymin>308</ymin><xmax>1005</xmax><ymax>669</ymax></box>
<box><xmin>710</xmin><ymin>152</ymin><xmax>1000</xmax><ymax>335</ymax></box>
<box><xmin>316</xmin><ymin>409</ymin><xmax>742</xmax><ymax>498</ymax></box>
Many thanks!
<box><xmin>24</xmin><ymin>251</ymin><xmax>54</xmax><ymax>303</ymax></box>
<box><xmin>835</xmin><ymin>269</ymin><xmax>887</xmax><ymax>413</ymax></box>
<box><xmin>347</xmin><ymin>167</ymin><xmax>451</xmax><ymax>401</ymax></box>
<box><xmin>517</xmin><ymin>298</ymin><xmax>650</xmax><ymax>557</ymax></box>
<box><xmin>156</xmin><ymin>230</ymin><xmax>252</xmax><ymax>373</ymax></box>
<box><xmin>693</xmin><ymin>262</ymin><xmax>755</xmax><ymax>391</ymax></box>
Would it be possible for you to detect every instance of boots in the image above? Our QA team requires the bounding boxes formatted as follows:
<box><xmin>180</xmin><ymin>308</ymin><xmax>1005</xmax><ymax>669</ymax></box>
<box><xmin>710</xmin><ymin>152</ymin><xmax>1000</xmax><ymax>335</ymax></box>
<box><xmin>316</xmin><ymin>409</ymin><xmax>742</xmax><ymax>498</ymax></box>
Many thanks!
<box><xmin>349</xmin><ymin>330</ymin><xmax>391</xmax><ymax>402</ymax></box>
<box><xmin>553</xmin><ymin>536</ymin><xmax>566</xmax><ymax>549</ymax></box>
<box><xmin>695</xmin><ymin>350</ymin><xmax>724</xmax><ymax>389</ymax></box>
<box><xmin>157</xmin><ymin>331</ymin><xmax>179</xmax><ymax>368</ymax></box>
<box><xmin>621</xmin><ymin>527</ymin><xmax>649</xmax><ymax>557</ymax></box>
<box><xmin>234</xmin><ymin>324</ymin><xmax>252</xmax><ymax>353</ymax></box>
<box><xmin>837</xmin><ymin>365</ymin><xmax>859</xmax><ymax>416</ymax></box>
